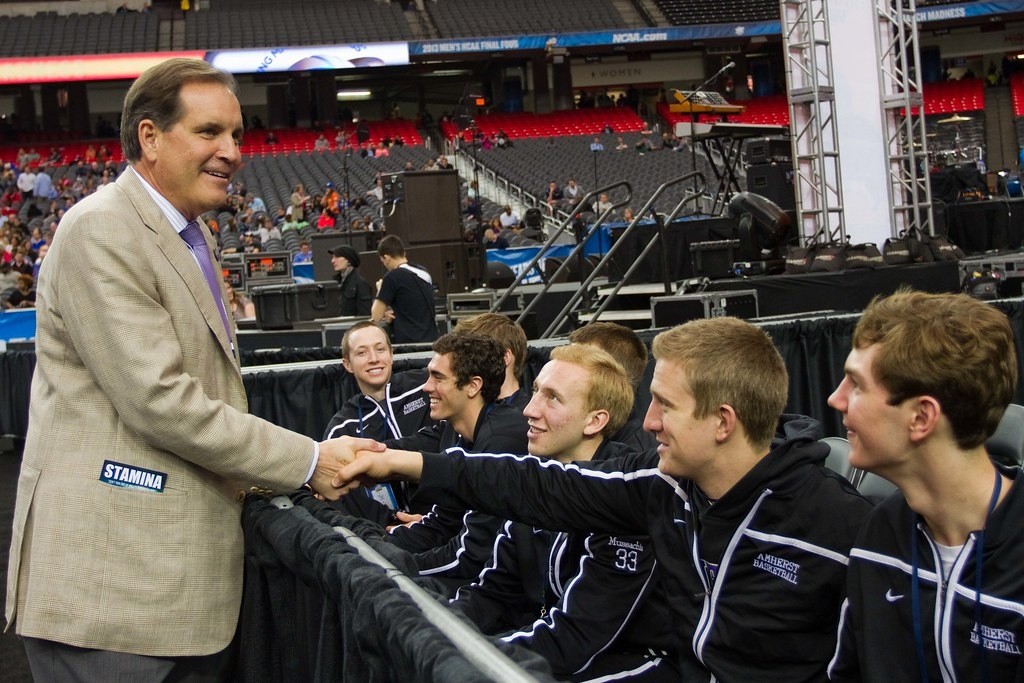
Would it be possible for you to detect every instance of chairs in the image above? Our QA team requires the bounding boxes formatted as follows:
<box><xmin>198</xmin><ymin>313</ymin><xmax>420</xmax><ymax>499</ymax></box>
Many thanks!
<box><xmin>1</xmin><ymin>0</ymin><xmax>1024</xmax><ymax>312</ymax></box>
<box><xmin>816</xmin><ymin>404</ymin><xmax>1024</xmax><ymax>508</ymax></box>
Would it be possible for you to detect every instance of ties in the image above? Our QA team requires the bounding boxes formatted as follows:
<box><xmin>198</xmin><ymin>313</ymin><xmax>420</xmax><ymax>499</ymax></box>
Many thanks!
<box><xmin>182</xmin><ymin>220</ymin><xmax>236</xmax><ymax>359</ymax></box>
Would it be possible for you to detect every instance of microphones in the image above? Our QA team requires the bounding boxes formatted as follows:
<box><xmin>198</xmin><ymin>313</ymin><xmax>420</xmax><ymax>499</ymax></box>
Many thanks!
<box><xmin>590</xmin><ymin>138</ymin><xmax>604</xmax><ymax>152</ymax></box>
<box><xmin>718</xmin><ymin>62</ymin><xmax>735</xmax><ymax>73</ymax></box>
<box><xmin>460</xmin><ymin>83</ymin><xmax>469</xmax><ymax>105</ymax></box>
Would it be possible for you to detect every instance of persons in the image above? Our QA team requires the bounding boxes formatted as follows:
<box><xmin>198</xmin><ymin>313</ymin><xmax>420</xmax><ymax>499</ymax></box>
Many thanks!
<box><xmin>314</xmin><ymin>290</ymin><xmax>1024</xmax><ymax>683</ymax></box>
<box><xmin>3</xmin><ymin>57</ymin><xmax>386</xmax><ymax>683</ymax></box>
<box><xmin>0</xmin><ymin>88</ymin><xmax>689</xmax><ymax>344</ymax></box>
<box><xmin>996</xmin><ymin>168</ymin><xmax>1024</xmax><ymax>198</ymax></box>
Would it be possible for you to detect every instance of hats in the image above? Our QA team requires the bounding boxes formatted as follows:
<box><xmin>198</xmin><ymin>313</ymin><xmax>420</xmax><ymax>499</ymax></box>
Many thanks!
<box><xmin>324</xmin><ymin>183</ymin><xmax>332</xmax><ymax>187</ymax></box>
<box><xmin>328</xmin><ymin>245</ymin><xmax>362</xmax><ymax>269</ymax></box>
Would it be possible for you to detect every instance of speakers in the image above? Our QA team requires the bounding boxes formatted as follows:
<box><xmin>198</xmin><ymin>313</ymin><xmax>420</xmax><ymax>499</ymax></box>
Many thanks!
<box><xmin>746</xmin><ymin>161</ymin><xmax>794</xmax><ymax>210</ymax></box>
<box><xmin>311</xmin><ymin>169</ymin><xmax>479</xmax><ymax>296</ymax></box>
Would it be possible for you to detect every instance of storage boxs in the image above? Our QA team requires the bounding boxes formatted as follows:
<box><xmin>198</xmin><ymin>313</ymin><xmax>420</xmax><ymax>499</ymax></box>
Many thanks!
<box><xmin>250</xmin><ymin>281</ymin><xmax>340</xmax><ymax>326</ymax></box>
